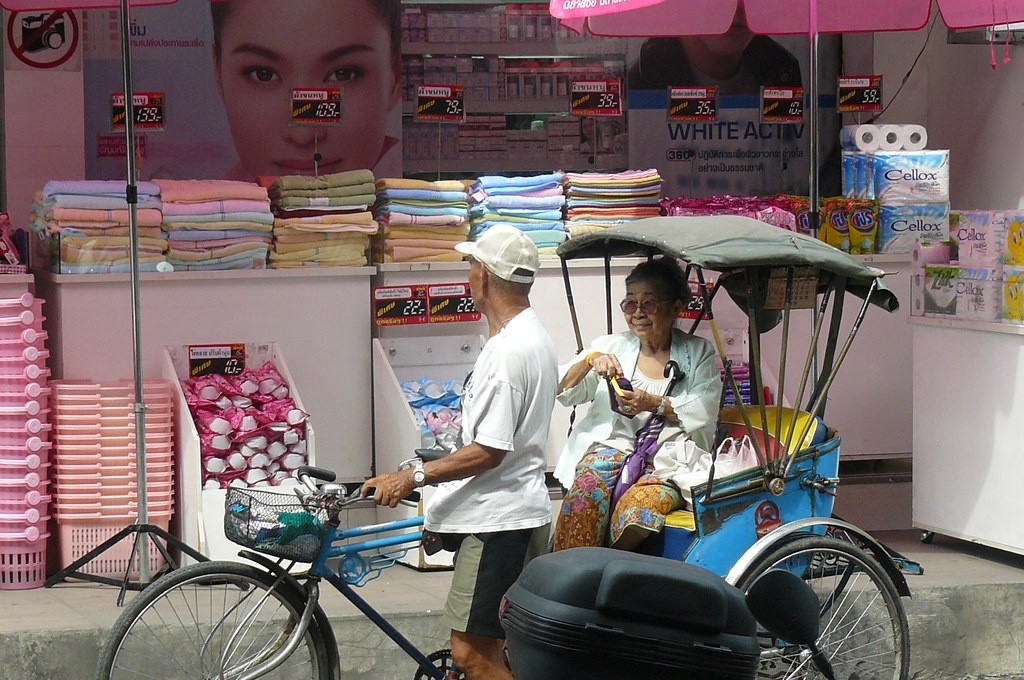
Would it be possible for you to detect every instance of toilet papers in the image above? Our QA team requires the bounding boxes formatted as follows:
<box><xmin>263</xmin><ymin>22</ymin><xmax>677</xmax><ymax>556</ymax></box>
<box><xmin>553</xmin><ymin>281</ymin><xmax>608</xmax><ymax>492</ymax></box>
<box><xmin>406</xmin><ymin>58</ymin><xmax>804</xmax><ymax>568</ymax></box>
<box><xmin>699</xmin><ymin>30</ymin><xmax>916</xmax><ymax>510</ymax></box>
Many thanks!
<box><xmin>838</xmin><ymin>122</ymin><xmax>1024</xmax><ymax>328</ymax></box>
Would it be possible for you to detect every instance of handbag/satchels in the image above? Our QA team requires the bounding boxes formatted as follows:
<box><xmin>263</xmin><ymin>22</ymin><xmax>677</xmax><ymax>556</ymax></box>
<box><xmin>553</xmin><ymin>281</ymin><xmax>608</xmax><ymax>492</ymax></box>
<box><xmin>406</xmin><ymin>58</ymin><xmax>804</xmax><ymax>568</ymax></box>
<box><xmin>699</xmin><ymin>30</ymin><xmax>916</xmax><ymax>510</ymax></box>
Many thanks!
<box><xmin>653</xmin><ymin>433</ymin><xmax>761</xmax><ymax>511</ymax></box>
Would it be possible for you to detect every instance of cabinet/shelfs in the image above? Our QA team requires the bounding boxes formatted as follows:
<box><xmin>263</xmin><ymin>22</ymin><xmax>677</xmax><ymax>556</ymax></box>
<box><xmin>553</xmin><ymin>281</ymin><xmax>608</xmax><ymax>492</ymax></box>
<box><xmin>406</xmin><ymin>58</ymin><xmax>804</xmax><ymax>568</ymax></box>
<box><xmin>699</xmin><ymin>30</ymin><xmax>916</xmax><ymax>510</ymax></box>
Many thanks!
<box><xmin>400</xmin><ymin>0</ymin><xmax>631</xmax><ymax>180</ymax></box>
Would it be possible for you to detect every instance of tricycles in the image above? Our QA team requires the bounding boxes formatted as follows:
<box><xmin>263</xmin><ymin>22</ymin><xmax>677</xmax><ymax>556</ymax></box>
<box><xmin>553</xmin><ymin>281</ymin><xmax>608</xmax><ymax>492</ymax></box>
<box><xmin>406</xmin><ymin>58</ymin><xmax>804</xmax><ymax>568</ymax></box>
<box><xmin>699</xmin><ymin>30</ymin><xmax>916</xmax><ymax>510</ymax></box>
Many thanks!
<box><xmin>99</xmin><ymin>228</ymin><xmax>926</xmax><ymax>680</ymax></box>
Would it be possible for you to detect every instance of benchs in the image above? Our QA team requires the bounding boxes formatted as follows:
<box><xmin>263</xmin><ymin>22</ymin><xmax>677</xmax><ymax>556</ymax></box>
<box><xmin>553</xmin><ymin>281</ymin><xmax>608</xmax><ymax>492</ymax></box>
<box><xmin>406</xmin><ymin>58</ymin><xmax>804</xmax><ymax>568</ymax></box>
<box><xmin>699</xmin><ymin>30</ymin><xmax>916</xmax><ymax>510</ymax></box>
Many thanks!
<box><xmin>662</xmin><ymin>406</ymin><xmax>826</xmax><ymax>561</ymax></box>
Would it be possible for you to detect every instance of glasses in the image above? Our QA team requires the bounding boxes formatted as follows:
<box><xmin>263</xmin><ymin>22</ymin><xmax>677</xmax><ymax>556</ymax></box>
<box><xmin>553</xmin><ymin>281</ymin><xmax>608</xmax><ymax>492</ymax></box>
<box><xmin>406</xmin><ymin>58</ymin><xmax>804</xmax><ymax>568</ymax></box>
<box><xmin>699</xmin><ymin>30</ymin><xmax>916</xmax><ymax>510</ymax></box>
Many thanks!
<box><xmin>619</xmin><ymin>297</ymin><xmax>678</xmax><ymax>317</ymax></box>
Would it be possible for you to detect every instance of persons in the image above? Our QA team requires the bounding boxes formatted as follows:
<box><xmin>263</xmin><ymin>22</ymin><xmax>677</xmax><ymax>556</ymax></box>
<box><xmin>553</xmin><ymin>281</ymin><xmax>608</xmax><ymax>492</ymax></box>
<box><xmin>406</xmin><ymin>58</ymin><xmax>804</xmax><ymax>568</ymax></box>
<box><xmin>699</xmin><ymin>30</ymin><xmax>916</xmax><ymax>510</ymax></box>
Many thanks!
<box><xmin>579</xmin><ymin>119</ymin><xmax>594</xmax><ymax>150</ymax></box>
<box><xmin>629</xmin><ymin>0</ymin><xmax>802</xmax><ymax>109</ymax></box>
<box><xmin>209</xmin><ymin>0</ymin><xmax>401</xmax><ymax>183</ymax></box>
<box><xmin>553</xmin><ymin>256</ymin><xmax>720</xmax><ymax>552</ymax></box>
<box><xmin>361</xmin><ymin>224</ymin><xmax>559</xmax><ymax>680</ymax></box>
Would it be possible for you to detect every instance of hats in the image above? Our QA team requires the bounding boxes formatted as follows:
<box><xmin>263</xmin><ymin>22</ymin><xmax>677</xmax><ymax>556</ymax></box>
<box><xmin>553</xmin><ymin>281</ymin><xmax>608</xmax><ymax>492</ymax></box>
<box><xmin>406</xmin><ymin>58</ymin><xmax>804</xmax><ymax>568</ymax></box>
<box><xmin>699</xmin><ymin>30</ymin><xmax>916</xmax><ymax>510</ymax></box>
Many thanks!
<box><xmin>455</xmin><ymin>223</ymin><xmax>540</xmax><ymax>285</ymax></box>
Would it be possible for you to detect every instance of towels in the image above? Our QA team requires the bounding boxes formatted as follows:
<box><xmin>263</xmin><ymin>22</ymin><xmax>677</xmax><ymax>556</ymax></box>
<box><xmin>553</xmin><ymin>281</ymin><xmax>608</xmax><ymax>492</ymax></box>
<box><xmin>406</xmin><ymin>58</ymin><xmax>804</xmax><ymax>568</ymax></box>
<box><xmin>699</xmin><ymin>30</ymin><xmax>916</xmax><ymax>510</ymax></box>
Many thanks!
<box><xmin>25</xmin><ymin>165</ymin><xmax>669</xmax><ymax>277</ymax></box>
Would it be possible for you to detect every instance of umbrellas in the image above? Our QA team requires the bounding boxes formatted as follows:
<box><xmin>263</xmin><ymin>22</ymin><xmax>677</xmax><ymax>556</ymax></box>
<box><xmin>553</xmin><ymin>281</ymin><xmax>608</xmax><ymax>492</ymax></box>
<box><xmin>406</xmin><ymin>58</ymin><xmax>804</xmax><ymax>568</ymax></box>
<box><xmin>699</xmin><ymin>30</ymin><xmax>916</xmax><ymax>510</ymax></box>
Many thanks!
<box><xmin>550</xmin><ymin>0</ymin><xmax>1024</xmax><ymax>392</ymax></box>
<box><xmin>0</xmin><ymin>0</ymin><xmax>178</xmax><ymax>594</ymax></box>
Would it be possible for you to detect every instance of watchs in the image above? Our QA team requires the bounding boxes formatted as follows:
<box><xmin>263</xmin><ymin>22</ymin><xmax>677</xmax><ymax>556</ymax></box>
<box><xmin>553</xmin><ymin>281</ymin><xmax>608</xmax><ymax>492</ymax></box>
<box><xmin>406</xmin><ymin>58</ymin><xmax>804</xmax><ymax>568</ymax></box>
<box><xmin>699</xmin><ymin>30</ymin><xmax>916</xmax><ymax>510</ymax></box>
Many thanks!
<box><xmin>413</xmin><ymin>463</ymin><xmax>425</xmax><ymax>487</ymax></box>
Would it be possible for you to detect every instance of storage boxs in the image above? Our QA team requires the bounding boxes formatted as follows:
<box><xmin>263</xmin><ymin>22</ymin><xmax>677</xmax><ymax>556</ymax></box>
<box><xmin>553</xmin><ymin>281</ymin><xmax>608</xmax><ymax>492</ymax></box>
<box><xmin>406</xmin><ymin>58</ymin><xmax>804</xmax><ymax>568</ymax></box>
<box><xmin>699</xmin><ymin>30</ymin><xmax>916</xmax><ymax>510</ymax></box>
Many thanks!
<box><xmin>0</xmin><ymin>262</ymin><xmax>174</xmax><ymax>591</ymax></box>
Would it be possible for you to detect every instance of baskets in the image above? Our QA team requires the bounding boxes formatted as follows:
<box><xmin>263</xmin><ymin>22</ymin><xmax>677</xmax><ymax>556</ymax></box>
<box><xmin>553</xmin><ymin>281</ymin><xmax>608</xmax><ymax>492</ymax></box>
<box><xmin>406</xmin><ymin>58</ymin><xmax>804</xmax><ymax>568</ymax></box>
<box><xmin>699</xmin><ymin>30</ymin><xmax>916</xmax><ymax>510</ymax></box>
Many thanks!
<box><xmin>223</xmin><ymin>486</ymin><xmax>335</xmax><ymax>564</ymax></box>
<box><xmin>0</xmin><ymin>265</ymin><xmax>176</xmax><ymax>586</ymax></box>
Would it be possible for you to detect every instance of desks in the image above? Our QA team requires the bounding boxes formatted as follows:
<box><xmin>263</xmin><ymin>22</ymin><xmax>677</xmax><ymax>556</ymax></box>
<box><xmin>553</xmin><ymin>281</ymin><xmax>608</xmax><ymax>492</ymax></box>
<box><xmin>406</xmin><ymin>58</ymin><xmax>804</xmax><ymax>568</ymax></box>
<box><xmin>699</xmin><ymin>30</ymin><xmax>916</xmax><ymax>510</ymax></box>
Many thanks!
<box><xmin>29</xmin><ymin>264</ymin><xmax>375</xmax><ymax>576</ymax></box>
<box><xmin>905</xmin><ymin>313</ymin><xmax>1024</xmax><ymax>558</ymax></box>
<box><xmin>375</xmin><ymin>253</ymin><xmax>918</xmax><ymax>579</ymax></box>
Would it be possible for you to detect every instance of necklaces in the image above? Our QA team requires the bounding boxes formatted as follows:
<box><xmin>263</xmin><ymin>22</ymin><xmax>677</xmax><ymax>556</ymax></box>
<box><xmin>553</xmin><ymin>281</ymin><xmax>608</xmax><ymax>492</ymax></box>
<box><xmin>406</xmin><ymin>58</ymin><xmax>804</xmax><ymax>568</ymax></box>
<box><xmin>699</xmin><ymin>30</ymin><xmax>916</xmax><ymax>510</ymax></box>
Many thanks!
<box><xmin>498</xmin><ymin>307</ymin><xmax>524</xmax><ymax>332</ymax></box>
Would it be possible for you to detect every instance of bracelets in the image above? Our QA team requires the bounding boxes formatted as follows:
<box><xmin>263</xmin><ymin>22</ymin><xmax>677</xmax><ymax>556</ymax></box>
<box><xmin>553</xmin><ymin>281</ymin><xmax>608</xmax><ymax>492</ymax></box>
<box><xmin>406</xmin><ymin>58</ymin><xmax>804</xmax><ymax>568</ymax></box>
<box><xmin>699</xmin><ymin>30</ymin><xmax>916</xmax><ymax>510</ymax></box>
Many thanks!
<box><xmin>586</xmin><ymin>351</ymin><xmax>597</xmax><ymax>368</ymax></box>
<box><xmin>656</xmin><ymin>397</ymin><xmax>664</xmax><ymax>415</ymax></box>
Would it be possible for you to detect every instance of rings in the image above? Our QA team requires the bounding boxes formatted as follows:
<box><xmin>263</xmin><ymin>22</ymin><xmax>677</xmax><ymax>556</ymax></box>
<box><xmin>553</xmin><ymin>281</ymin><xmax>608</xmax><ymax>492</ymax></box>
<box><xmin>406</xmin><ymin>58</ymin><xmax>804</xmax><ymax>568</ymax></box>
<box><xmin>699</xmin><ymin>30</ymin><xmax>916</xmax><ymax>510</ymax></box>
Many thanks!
<box><xmin>623</xmin><ymin>406</ymin><xmax>631</xmax><ymax>412</ymax></box>
